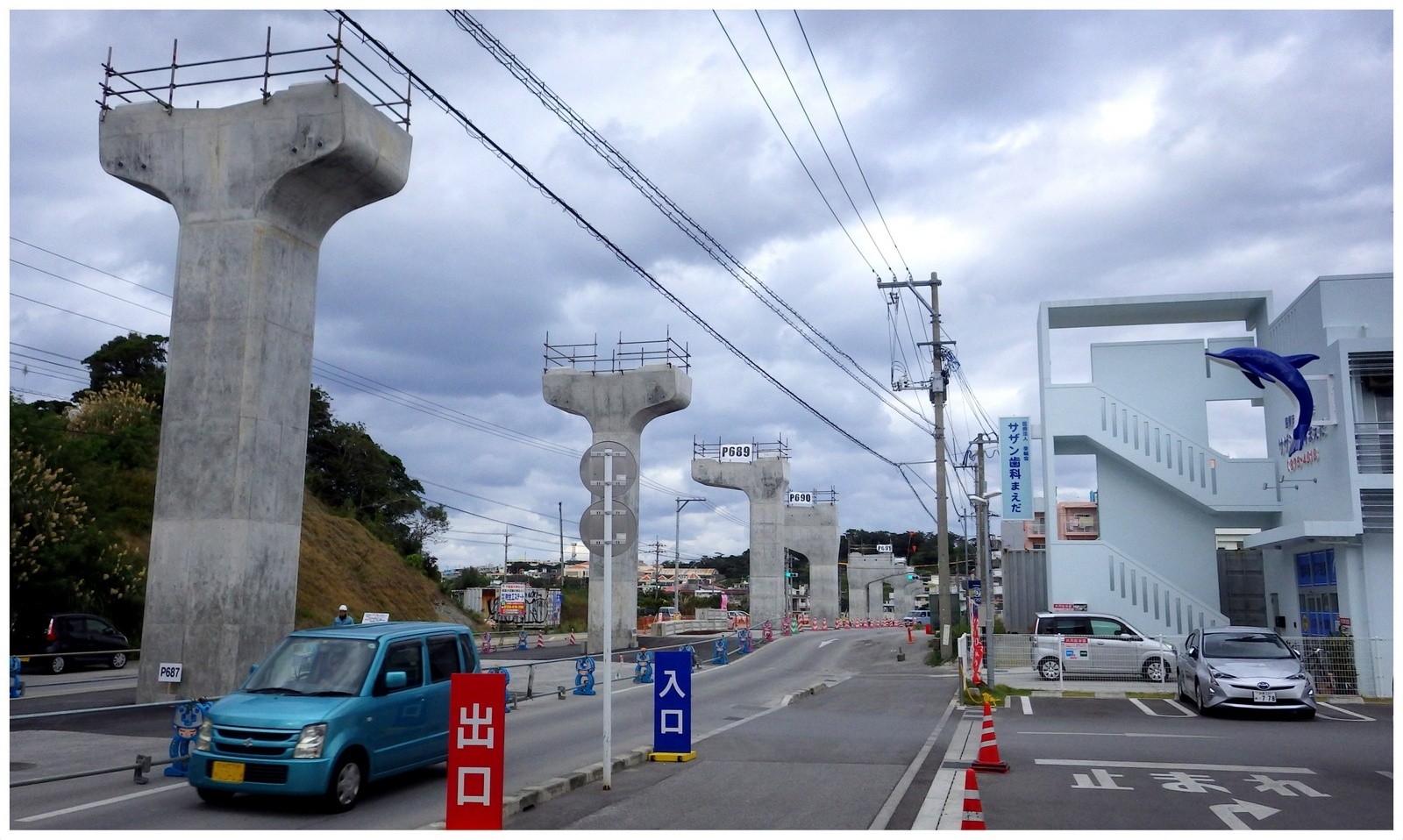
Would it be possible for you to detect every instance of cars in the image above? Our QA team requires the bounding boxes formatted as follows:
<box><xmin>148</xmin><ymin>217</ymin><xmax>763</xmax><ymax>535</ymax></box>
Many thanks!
<box><xmin>1178</xmin><ymin>626</ymin><xmax>1318</xmax><ymax>721</ymax></box>
<box><xmin>727</xmin><ymin>611</ymin><xmax>750</xmax><ymax>629</ymax></box>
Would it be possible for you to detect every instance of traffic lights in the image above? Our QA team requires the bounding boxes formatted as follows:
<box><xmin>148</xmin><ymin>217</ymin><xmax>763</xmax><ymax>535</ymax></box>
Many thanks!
<box><xmin>785</xmin><ymin>572</ymin><xmax>798</xmax><ymax>577</ymax></box>
<box><xmin>908</xmin><ymin>575</ymin><xmax>920</xmax><ymax>581</ymax></box>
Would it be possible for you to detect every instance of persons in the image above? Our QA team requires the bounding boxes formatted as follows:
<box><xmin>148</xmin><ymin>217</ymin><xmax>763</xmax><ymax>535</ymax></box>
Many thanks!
<box><xmin>334</xmin><ymin>604</ymin><xmax>354</xmax><ymax>625</ymax></box>
<box><xmin>323</xmin><ymin>653</ymin><xmax>365</xmax><ymax>687</ymax></box>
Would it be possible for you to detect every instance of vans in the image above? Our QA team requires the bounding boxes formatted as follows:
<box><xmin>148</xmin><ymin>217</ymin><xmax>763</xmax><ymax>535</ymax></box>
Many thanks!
<box><xmin>187</xmin><ymin>621</ymin><xmax>480</xmax><ymax>814</ymax></box>
<box><xmin>10</xmin><ymin>613</ymin><xmax>128</xmax><ymax>675</ymax></box>
<box><xmin>1030</xmin><ymin>612</ymin><xmax>1177</xmax><ymax>683</ymax></box>
<box><xmin>659</xmin><ymin>606</ymin><xmax>681</xmax><ymax>619</ymax></box>
<box><xmin>883</xmin><ymin>604</ymin><xmax>895</xmax><ymax>620</ymax></box>
<box><xmin>903</xmin><ymin>610</ymin><xmax>930</xmax><ymax>627</ymax></box>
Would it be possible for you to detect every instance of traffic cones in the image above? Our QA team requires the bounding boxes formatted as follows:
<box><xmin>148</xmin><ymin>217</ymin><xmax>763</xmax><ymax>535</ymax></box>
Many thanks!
<box><xmin>970</xmin><ymin>703</ymin><xmax>1010</xmax><ymax>774</ymax></box>
<box><xmin>961</xmin><ymin>769</ymin><xmax>986</xmax><ymax>829</ymax></box>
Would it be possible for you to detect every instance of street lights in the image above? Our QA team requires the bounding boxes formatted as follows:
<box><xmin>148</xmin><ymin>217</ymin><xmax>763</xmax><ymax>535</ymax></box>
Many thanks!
<box><xmin>968</xmin><ymin>489</ymin><xmax>1003</xmax><ymax>691</ymax></box>
